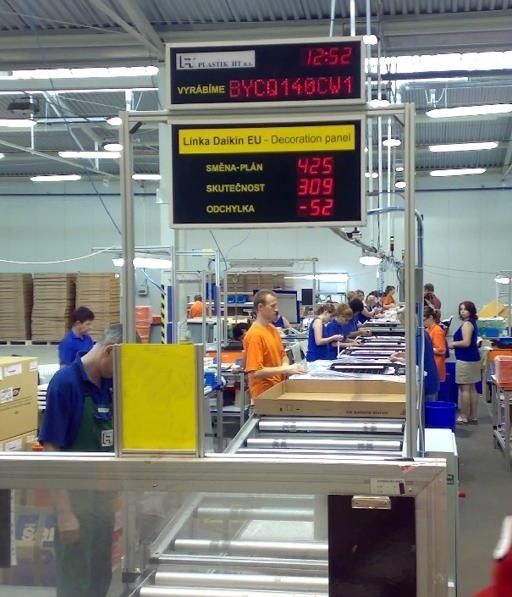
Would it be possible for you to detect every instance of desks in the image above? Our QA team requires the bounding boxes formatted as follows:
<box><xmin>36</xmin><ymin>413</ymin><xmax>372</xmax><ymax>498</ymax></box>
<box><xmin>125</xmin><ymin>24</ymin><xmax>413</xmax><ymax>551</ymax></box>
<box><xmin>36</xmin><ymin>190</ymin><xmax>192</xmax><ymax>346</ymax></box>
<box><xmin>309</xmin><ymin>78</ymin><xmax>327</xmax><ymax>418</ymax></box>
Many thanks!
<box><xmin>203</xmin><ymin>381</ymin><xmax>223</xmax><ymax>453</ymax></box>
<box><xmin>221</xmin><ymin>372</ymin><xmax>246</xmax><ymax>429</ymax></box>
<box><xmin>491</xmin><ymin>375</ymin><xmax>512</xmax><ymax>473</ymax></box>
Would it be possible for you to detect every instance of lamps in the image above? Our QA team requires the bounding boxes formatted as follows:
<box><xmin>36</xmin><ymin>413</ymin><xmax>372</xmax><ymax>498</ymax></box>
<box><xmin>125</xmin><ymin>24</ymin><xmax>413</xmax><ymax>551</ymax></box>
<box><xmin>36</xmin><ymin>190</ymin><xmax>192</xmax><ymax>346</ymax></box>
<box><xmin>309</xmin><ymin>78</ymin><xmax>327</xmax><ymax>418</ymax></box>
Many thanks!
<box><xmin>0</xmin><ymin>67</ymin><xmax>161</xmax><ymax>182</ymax></box>
<box><xmin>304</xmin><ymin>273</ymin><xmax>349</xmax><ymax>281</ymax></box>
<box><xmin>359</xmin><ymin>252</ymin><xmax>382</xmax><ymax>266</ymax></box>
<box><xmin>495</xmin><ymin>275</ymin><xmax>510</xmax><ymax>285</ymax></box>
<box><xmin>365</xmin><ymin>50</ymin><xmax>512</xmax><ymax>188</ymax></box>
<box><xmin>112</xmin><ymin>251</ymin><xmax>172</xmax><ymax>269</ymax></box>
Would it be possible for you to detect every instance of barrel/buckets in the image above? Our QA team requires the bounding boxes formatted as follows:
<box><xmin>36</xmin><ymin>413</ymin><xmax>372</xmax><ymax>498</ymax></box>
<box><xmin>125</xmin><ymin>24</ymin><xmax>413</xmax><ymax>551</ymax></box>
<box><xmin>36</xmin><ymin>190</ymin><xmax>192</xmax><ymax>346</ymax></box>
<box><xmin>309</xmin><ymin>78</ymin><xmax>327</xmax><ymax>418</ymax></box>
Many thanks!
<box><xmin>438</xmin><ymin>362</ymin><xmax>458</xmax><ymax>403</ymax></box>
<box><xmin>425</xmin><ymin>400</ymin><xmax>458</xmax><ymax>434</ymax></box>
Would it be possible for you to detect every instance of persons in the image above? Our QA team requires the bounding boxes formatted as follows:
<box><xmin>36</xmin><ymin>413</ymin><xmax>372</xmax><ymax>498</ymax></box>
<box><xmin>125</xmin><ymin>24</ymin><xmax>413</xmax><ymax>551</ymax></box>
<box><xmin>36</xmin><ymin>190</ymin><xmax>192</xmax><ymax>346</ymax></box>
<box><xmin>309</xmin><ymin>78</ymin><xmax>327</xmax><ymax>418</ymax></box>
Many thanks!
<box><xmin>189</xmin><ymin>295</ymin><xmax>207</xmax><ymax>318</ymax></box>
<box><xmin>38</xmin><ymin>323</ymin><xmax>142</xmax><ymax>596</ymax></box>
<box><xmin>58</xmin><ymin>305</ymin><xmax>94</xmax><ymax>368</ymax></box>
<box><xmin>231</xmin><ymin>284</ymin><xmax>484</xmax><ymax>426</ymax></box>
